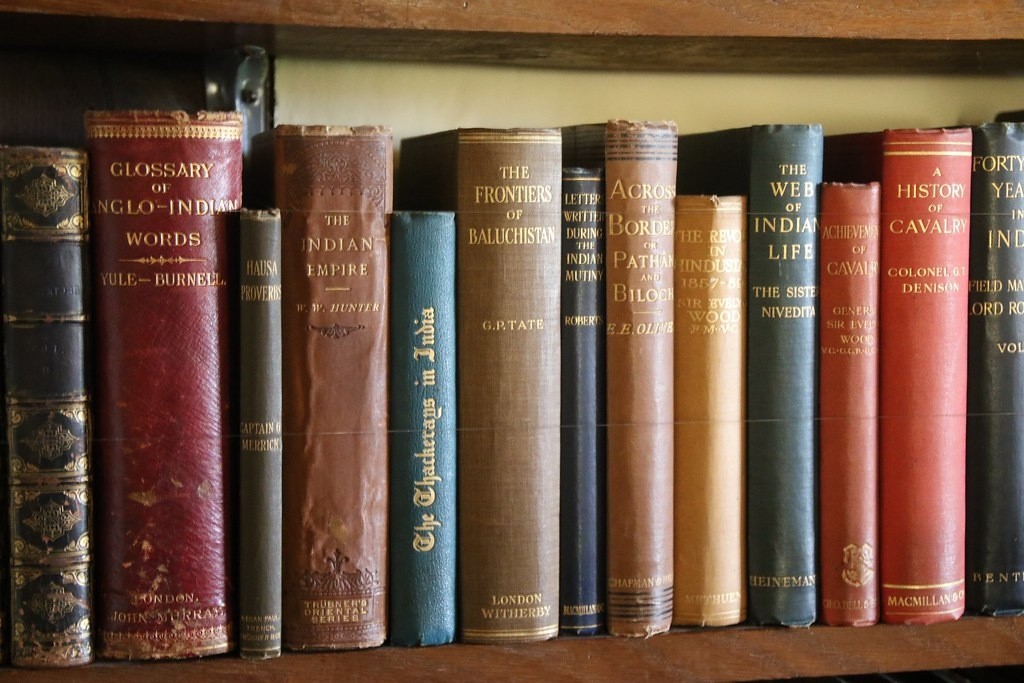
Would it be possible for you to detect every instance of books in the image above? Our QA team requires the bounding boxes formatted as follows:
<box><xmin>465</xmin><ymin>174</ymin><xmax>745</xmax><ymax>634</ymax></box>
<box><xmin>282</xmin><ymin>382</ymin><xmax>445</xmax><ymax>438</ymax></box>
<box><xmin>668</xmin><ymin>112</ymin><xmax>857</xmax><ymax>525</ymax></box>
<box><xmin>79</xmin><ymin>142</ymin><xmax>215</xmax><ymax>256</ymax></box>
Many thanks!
<box><xmin>0</xmin><ymin>109</ymin><xmax>1024</xmax><ymax>669</ymax></box>
